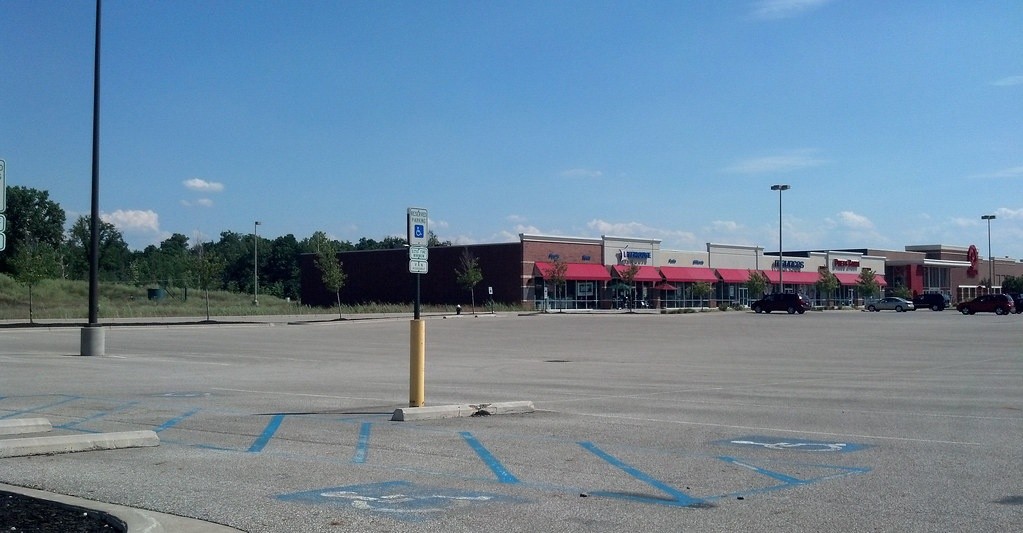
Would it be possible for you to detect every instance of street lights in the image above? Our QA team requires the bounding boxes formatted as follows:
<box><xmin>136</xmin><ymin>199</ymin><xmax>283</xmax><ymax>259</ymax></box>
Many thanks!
<box><xmin>254</xmin><ymin>221</ymin><xmax>261</xmax><ymax>303</ymax></box>
<box><xmin>771</xmin><ymin>185</ymin><xmax>791</xmax><ymax>293</ymax></box>
<box><xmin>981</xmin><ymin>215</ymin><xmax>996</xmax><ymax>294</ymax></box>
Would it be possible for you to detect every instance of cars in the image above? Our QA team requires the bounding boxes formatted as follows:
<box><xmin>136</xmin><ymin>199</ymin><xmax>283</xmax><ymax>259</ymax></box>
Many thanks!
<box><xmin>865</xmin><ymin>297</ymin><xmax>914</xmax><ymax>312</ymax></box>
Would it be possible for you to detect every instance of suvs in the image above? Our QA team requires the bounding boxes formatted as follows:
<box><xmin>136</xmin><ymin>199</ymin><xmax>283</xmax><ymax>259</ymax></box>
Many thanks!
<box><xmin>957</xmin><ymin>294</ymin><xmax>1014</xmax><ymax>316</ymax></box>
<box><xmin>910</xmin><ymin>293</ymin><xmax>951</xmax><ymax>311</ymax></box>
<box><xmin>751</xmin><ymin>293</ymin><xmax>812</xmax><ymax>314</ymax></box>
